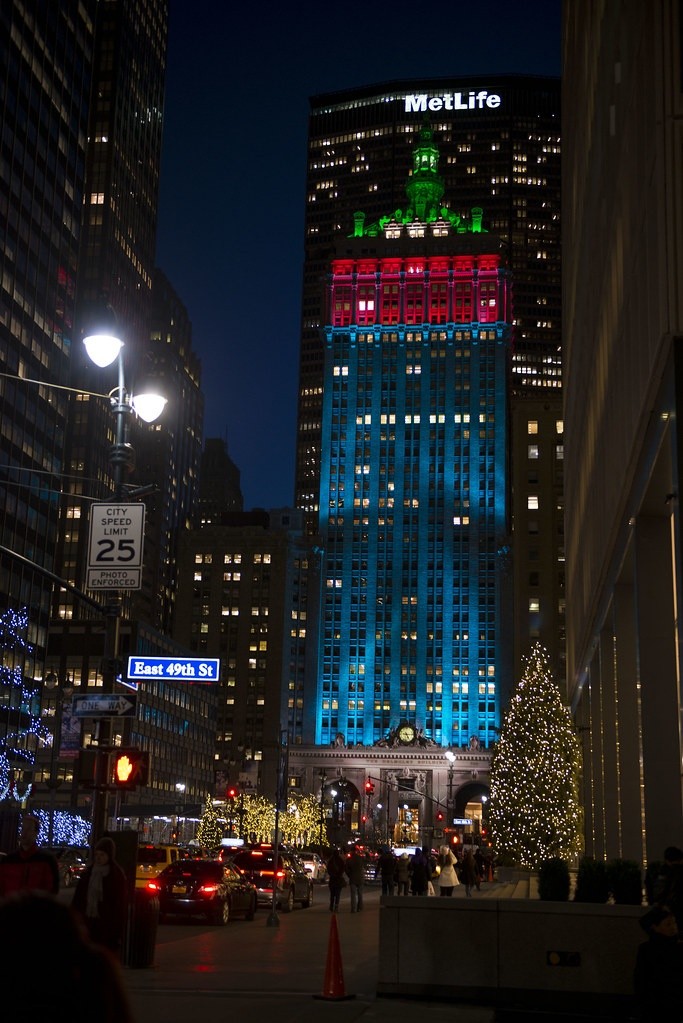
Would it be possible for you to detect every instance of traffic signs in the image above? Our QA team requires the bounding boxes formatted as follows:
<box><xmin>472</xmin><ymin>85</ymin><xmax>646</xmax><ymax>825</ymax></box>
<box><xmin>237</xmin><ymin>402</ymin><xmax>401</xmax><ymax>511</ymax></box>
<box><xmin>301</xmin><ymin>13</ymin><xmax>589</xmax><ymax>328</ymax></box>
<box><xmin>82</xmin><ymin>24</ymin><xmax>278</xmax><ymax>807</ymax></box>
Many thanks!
<box><xmin>71</xmin><ymin>693</ymin><xmax>137</xmax><ymax>720</ymax></box>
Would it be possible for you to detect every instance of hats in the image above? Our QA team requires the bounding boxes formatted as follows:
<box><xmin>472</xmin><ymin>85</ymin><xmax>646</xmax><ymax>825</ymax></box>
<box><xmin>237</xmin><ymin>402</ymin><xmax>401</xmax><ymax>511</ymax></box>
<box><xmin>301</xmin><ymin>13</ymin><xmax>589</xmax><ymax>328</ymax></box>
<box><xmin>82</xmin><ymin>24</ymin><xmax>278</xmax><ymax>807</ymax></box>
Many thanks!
<box><xmin>440</xmin><ymin>845</ymin><xmax>449</xmax><ymax>856</ymax></box>
<box><xmin>95</xmin><ymin>837</ymin><xmax>115</xmax><ymax>858</ymax></box>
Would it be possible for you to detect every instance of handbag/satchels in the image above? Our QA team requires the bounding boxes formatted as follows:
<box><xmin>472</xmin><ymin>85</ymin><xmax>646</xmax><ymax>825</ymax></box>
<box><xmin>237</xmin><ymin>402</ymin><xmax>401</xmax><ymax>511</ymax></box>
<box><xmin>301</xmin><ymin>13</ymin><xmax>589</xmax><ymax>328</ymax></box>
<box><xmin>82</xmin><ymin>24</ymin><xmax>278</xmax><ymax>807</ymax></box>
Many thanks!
<box><xmin>393</xmin><ymin>871</ymin><xmax>398</xmax><ymax>883</ymax></box>
<box><xmin>338</xmin><ymin>877</ymin><xmax>347</xmax><ymax>888</ymax></box>
<box><xmin>427</xmin><ymin>881</ymin><xmax>436</xmax><ymax>896</ymax></box>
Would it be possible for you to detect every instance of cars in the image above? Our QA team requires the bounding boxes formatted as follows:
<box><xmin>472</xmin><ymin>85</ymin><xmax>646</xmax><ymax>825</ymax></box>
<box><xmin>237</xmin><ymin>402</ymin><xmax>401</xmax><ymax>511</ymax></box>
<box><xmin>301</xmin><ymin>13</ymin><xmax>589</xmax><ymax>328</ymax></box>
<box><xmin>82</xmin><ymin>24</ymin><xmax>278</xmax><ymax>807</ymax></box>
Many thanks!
<box><xmin>229</xmin><ymin>850</ymin><xmax>315</xmax><ymax>913</ymax></box>
<box><xmin>296</xmin><ymin>851</ymin><xmax>328</xmax><ymax>883</ymax></box>
<box><xmin>136</xmin><ymin>841</ymin><xmax>193</xmax><ymax>893</ymax></box>
<box><xmin>146</xmin><ymin>858</ymin><xmax>259</xmax><ymax>926</ymax></box>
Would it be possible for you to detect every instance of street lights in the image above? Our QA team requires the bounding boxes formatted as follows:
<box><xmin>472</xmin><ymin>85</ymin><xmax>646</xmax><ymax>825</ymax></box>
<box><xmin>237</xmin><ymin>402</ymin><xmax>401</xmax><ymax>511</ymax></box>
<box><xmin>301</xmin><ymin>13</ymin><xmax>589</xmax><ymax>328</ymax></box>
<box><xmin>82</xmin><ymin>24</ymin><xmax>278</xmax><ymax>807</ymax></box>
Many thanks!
<box><xmin>446</xmin><ymin>749</ymin><xmax>456</xmax><ymax>857</ymax></box>
<box><xmin>60</xmin><ymin>299</ymin><xmax>173</xmax><ymax>940</ymax></box>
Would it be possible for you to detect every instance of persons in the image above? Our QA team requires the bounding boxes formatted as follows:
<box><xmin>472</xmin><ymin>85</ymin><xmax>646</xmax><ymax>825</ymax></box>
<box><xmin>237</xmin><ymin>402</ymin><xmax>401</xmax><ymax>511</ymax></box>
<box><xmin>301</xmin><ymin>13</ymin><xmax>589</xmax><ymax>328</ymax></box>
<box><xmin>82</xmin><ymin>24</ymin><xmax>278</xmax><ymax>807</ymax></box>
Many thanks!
<box><xmin>0</xmin><ymin>815</ymin><xmax>682</xmax><ymax>1022</ymax></box>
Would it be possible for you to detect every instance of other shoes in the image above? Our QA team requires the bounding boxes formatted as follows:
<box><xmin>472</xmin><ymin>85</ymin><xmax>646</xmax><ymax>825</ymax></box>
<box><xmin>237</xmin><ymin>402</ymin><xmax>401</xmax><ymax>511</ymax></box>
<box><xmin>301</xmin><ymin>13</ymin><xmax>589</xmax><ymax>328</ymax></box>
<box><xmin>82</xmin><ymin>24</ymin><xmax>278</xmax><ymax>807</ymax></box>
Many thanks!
<box><xmin>351</xmin><ymin>908</ymin><xmax>361</xmax><ymax>913</ymax></box>
<box><xmin>329</xmin><ymin>903</ymin><xmax>338</xmax><ymax>913</ymax></box>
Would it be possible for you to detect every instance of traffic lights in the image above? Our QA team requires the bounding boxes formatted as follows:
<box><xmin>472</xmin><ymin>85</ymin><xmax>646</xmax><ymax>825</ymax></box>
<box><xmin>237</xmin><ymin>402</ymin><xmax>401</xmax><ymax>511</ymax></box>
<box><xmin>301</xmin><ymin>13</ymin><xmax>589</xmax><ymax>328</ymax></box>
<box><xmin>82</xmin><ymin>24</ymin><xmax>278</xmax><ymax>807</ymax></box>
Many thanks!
<box><xmin>365</xmin><ymin>782</ymin><xmax>375</xmax><ymax>796</ymax></box>
<box><xmin>437</xmin><ymin>811</ymin><xmax>443</xmax><ymax>822</ymax></box>
<box><xmin>106</xmin><ymin>746</ymin><xmax>140</xmax><ymax>789</ymax></box>
